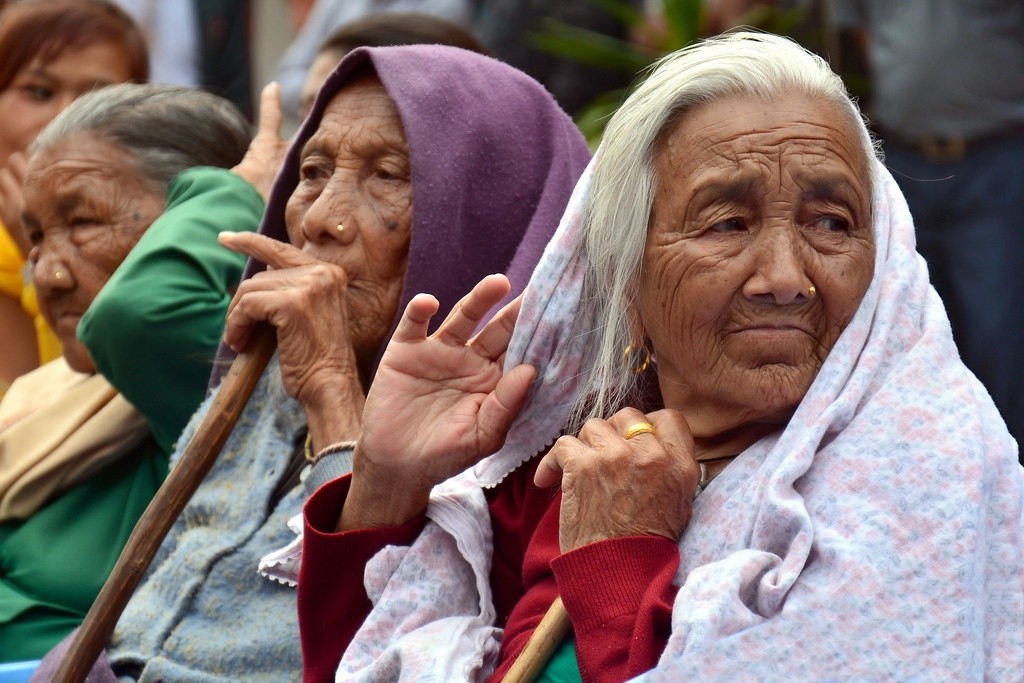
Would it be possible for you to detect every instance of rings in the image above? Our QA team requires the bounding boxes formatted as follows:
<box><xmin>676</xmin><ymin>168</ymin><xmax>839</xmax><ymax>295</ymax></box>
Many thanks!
<box><xmin>624</xmin><ymin>423</ymin><xmax>656</xmax><ymax>440</ymax></box>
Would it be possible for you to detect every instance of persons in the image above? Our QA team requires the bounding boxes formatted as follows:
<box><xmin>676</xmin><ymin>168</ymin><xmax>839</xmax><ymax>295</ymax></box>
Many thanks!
<box><xmin>1</xmin><ymin>80</ymin><xmax>291</xmax><ymax>672</ymax></box>
<box><xmin>296</xmin><ymin>11</ymin><xmax>491</xmax><ymax>137</ymax></box>
<box><xmin>31</xmin><ymin>44</ymin><xmax>592</xmax><ymax>683</ymax></box>
<box><xmin>0</xmin><ymin>0</ymin><xmax>152</xmax><ymax>402</ymax></box>
<box><xmin>297</xmin><ymin>29</ymin><xmax>1024</xmax><ymax>683</ymax></box>
